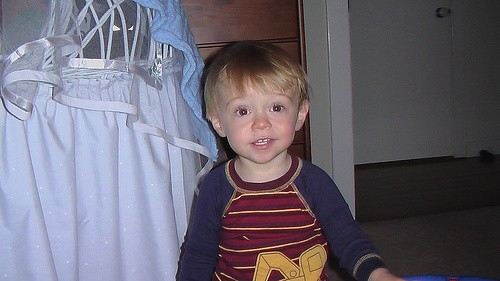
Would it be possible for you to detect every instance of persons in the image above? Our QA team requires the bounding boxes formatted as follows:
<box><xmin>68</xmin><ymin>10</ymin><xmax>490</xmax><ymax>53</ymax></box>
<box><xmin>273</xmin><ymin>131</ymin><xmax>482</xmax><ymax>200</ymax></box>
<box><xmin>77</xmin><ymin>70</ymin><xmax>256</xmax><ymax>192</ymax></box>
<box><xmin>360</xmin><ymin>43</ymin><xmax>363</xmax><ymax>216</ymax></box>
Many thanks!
<box><xmin>175</xmin><ymin>39</ymin><xmax>405</xmax><ymax>281</ymax></box>
